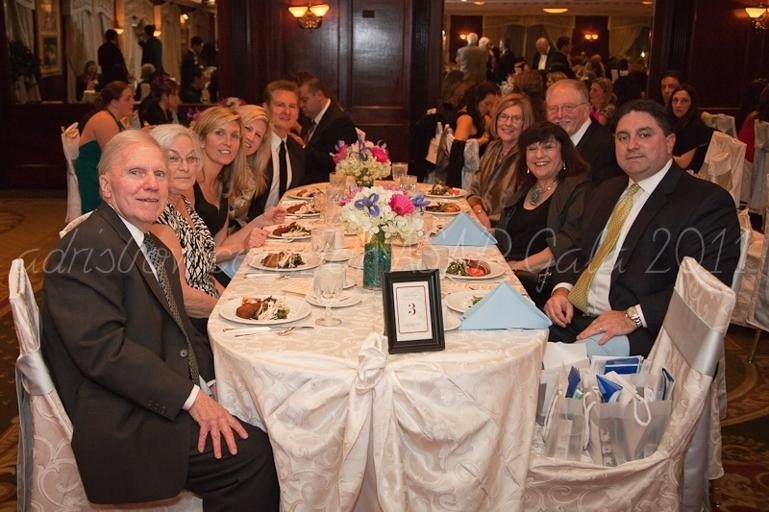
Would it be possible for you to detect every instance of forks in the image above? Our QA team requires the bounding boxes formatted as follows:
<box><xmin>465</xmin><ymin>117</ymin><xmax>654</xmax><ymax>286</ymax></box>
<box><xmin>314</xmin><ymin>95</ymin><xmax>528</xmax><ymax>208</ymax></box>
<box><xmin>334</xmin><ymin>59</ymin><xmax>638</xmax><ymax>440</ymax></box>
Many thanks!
<box><xmin>234</xmin><ymin>327</ymin><xmax>297</xmax><ymax>337</ymax></box>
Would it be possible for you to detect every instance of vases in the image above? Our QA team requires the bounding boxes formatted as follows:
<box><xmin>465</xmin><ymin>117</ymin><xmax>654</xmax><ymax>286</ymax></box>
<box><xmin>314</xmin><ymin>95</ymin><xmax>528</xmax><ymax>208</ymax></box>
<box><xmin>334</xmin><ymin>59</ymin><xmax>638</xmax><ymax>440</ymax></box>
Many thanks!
<box><xmin>362</xmin><ymin>241</ymin><xmax>393</xmax><ymax>291</ymax></box>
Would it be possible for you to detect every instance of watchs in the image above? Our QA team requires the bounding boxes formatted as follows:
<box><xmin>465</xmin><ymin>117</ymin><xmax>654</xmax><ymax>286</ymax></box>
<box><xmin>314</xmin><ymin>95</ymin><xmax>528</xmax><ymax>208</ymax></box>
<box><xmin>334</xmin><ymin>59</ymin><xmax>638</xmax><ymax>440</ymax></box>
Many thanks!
<box><xmin>623</xmin><ymin>305</ymin><xmax>643</xmax><ymax>329</ymax></box>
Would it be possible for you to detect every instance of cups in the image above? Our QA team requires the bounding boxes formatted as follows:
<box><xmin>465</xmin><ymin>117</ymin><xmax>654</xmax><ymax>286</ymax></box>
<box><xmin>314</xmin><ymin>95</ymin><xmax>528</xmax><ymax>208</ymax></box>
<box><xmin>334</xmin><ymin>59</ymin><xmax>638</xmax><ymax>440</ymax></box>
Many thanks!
<box><xmin>435</xmin><ymin>247</ymin><xmax>449</xmax><ymax>279</ymax></box>
<box><xmin>400</xmin><ymin>176</ymin><xmax>417</xmax><ymax>197</ymax></box>
<box><xmin>391</xmin><ymin>163</ymin><xmax>408</xmax><ymax>185</ymax></box>
<box><xmin>321</xmin><ymin>229</ymin><xmax>333</xmax><ymax>251</ymax></box>
<box><xmin>328</xmin><ymin>172</ymin><xmax>346</xmax><ymax>191</ymax></box>
<box><xmin>334</xmin><ymin>226</ymin><xmax>347</xmax><ymax>251</ymax></box>
<box><xmin>311</xmin><ymin>266</ymin><xmax>345</xmax><ymax>328</ymax></box>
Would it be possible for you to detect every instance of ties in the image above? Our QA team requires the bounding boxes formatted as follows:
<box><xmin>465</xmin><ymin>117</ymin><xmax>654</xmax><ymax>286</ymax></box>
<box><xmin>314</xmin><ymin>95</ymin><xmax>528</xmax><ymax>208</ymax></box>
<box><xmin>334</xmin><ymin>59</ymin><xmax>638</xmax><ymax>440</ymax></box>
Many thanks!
<box><xmin>568</xmin><ymin>182</ymin><xmax>640</xmax><ymax>316</ymax></box>
<box><xmin>279</xmin><ymin>140</ymin><xmax>288</xmax><ymax>201</ymax></box>
<box><xmin>142</xmin><ymin>231</ymin><xmax>200</xmax><ymax>396</ymax></box>
<box><xmin>303</xmin><ymin>120</ymin><xmax>315</xmax><ymax>147</ymax></box>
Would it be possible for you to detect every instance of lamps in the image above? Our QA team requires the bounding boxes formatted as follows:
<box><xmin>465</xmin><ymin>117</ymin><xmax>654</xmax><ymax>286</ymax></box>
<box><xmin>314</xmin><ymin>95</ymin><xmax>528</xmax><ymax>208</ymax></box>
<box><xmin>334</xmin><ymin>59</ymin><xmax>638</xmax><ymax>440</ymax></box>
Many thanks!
<box><xmin>290</xmin><ymin>0</ymin><xmax>330</xmax><ymax>32</ymax></box>
<box><xmin>544</xmin><ymin>6</ymin><xmax>572</xmax><ymax>16</ymax></box>
<box><xmin>582</xmin><ymin>29</ymin><xmax>605</xmax><ymax>44</ymax></box>
<box><xmin>730</xmin><ymin>0</ymin><xmax>767</xmax><ymax>30</ymax></box>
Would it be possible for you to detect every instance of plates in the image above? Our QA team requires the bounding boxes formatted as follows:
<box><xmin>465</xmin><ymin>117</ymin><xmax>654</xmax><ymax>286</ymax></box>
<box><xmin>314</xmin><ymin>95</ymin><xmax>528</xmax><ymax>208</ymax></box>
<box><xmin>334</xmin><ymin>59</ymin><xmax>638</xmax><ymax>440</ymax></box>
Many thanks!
<box><xmin>424</xmin><ymin>188</ymin><xmax>467</xmax><ymax>199</ymax></box>
<box><xmin>245</xmin><ymin>248</ymin><xmax>322</xmax><ymax>272</ymax></box>
<box><xmin>444</xmin><ymin>289</ymin><xmax>536</xmax><ymax>314</ymax></box>
<box><xmin>444</xmin><ymin>316</ymin><xmax>461</xmax><ymax>331</ymax></box>
<box><xmin>287</xmin><ymin>190</ymin><xmax>329</xmax><ymax>200</ymax></box>
<box><xmin>266</xmin><ymin>233</ymin><xmax>312</xmax><ymax>240</ymax></box>
<box><xmin>305</xmin><ymin>293</ymin><xmax>363</xmax><ymax>308</ymax></box>
<box><xmin>284</xmin><ymin>212</ymin><xmax>322</xmax><ymax>217</ymax></box>
<box><xmin>217</xmin><ymin>293</ymin><xmax>313</xmax><ymax>327</ymax></box>
<box><xmin>420</xmin><ymin>200</ymin><xmax>465</xmax><ymax>216</ymax></box>
<box><xmin>324</xmin><ymin>251</ymin><xmax>350</xmax><ymax>262</ymax></box>
<box><xmin>443</xmin><ymin>262</ymin><xmax>505</xmax><ymax>280</ymax></box>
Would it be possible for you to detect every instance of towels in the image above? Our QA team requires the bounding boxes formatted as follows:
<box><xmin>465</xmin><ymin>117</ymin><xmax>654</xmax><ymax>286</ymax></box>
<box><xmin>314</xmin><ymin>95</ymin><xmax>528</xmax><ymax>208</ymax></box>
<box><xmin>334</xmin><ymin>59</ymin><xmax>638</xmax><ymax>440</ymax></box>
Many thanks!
<box><xmin>456</xmin><ymin>280</ymin><xmax>554</xmax><ymax>331</ymax></box>
<box><xmin>430</xmin><ymin>212</ymin><xmax>498</xmax><ymax>252</ymax></box>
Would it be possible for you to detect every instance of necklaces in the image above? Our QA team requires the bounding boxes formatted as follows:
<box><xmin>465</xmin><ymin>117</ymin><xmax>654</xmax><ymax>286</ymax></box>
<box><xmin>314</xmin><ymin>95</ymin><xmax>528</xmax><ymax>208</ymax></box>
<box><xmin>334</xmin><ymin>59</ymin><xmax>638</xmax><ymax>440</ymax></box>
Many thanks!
<box><xmin>498</xmin><ymin>145</ymin><xmax>507</xmax><ymax>166</ymax></box>
<box><xmin>527</xmin><ymin>179</ymin><xmax>559</xmax><ymax>206</ymax></box>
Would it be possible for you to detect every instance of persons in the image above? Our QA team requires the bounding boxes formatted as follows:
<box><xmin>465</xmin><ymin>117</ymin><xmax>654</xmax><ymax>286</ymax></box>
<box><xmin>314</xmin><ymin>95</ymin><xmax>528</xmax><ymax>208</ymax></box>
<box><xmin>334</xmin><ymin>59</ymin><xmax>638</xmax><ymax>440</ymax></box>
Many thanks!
<box><xmin>180</xmin><ymin>35</ymin><xmax>217</xmax><ymax>103</ymax></box>
<box><xmin>509</xmin><ymin>68</ymin><xmax>570</xmax><ymax>97</ymax></box>
<box><xmin>542</xmin><ymin>98</ymin><xmax>741</xmax><ymax>359</ymax></box>
<box><xmin>147</xmin><ymin>123</ymin><xmax>227</xmax><ymax>320</ymax></box>
<box><xmin>137</xmin><ymin>81</ymin><xmax>180</xmax><ymax>126</ymax></box>
<box><xmin>666</xmin><ymin>85</ymin><xmax>709</xmax><ymax>174</ymax></box>
<box><xmin>605</xmin><ymin>56</ymin><xmax>646</xmax><ymax>101</ymax></box>
<box><xmin>445</xmin><ymin>80</ymin><xmax>497</xmax><ymax>187</ymax></box>
<box><xmin>146</xmin><ymin>72</ymin><xmax>174</xmax><ymax>96</ymax></box>
<box><xmin>479</xmin><ymin>37</ymin><xmax>516</xmax><ymax>84</ymax></box>
<box><xmin>75</xmin><ymin>81</ymin><xmax>134</xmax><ymax>215</ymax></box>
<box><xmin>248</xmin><ymin>80</ymin><xmax>306</xmax><ymax>220</ymax></box>
<box><xmin>229</xmin><ymin>104</ymin><xmax>274</xmax><ymax>235</ymax></box>
<box><xmin>134</xmin><ymin>64</ymin><xmax>156</xmax><ymax>101</ymax></box>
<box><xmin>290</xmin><ymin>75</ymin><xmax>358</xmax><ymax>188</ymax></box>
<box><xmin>455</xmin><ymin>31</ymin><xmax>486</xmax><ymax>80</ymax></box>
<box><xmin>542</xmin><ymin>78</ymin><xmax>622</xmax><ymax>184</ymax></box>
<box><xmin>466</xmin><ymin>92</ymin><xmax>538</xmax><ymax>228</ymax></box>
<box><xmin>660</xmin><ymin>75</ymin><xmax>679</xmax><ymax>107</ymax></box>
<box><xmin>548</xmin><ymin>35</ymin><xmax>577</xmax><ymax>79</ymax></box>
<box><xmin>532</xmin><ymin>37</ymin><xmax>576</xmax><ymax>80</ymax></box>
<box><xmin>37</xmin><ymin>128</ymin><xmax>281</xmax><ymax>510</ymax></box>
<box><xmin>136</xmin><ymin>24</ymin><xmax>165</xmax><ymax>75</ymax></box>
<box><xmin>98</xmin><ymin>29</ymin><xmax>130</xmax><ymax>92</ymax></box>
<box><xmin>582</xmin><ymin>54</ymin><xmax>605</xmax><ymax>78</ymax></box>
<box><xmin>589</xmin><ymin>77</ymin><xmax>617</xmax><ymax>126</ymax></box>
<box><xmin>188</xmin><ymin>105</ymin><xmax>288</xmax><ymax>259</ymax></box>
<box><xmin>76</xmin><ymin>61</ymin><xmax>107</xmax><ymax>102</ymax></box>
<box><xmin>494</xmin><ymin>123</ymin><xmax>597</xmax><ymax>307</ymax></box>
<box><xmin>734</xmin><ymin>76</ymin><xmax>769</xmax><ymax>165</ymax></box>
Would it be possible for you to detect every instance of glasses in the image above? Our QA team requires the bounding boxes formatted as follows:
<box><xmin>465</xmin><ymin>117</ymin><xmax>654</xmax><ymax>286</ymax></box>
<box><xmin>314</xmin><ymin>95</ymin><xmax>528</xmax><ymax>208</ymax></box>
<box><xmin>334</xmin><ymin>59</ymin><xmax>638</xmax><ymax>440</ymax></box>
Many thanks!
<box><xmin>542</xmin><ymin>102</ymin><xmax>591</xmax><ymax>113</ymax></box>
<box><xmin>498</xmin><ymin>113</ymin><xmax>526</xmax><ymax>123</ymax></box>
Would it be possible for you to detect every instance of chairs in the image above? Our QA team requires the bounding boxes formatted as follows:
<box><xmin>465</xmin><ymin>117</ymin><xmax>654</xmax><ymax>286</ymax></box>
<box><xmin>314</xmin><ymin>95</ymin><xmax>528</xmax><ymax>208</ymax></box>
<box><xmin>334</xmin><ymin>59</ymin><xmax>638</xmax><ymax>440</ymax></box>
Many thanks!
<box><xmin>750</xmin><ymin>120</ymin><xmax>769</xmax><ymax>211</ymax></box>
<box><xmin>731</xmin><ymin>209</ymin><xmax>747</xmax><ymax>291</ymax></box>
<box><xmin>714</xmin><ymin>114</ymin><xmax>734</xmax><ymax>139</ymax></box>
<box><xmin>445</xmin><ymin>133</ymin><xmax>478</xmax><ymax>192</ymax></box>
<box><xmin>61</xmin><ymin>121</ymin><xmax>83</xmax><ymax>224</ymax></box>
<box><xmin>426</xmin><ymin>122</ymin><xmax>449</xmax><ymax>163</ymax></box>
<box><xmin>57</xmin><ymin>207</ymin><xmax>93</xmax><ymax>235</ymax></box>
<box><xmin>748</xmin><ymin>208</ymin><xmax>769</xmax><ymax>363</ymax></box>
<box><xmin>699</xmin><ymin>112</ymin><xmax>713</xmax><ymax>128</ymax></box>
<box><xmin>527</xmin><ymin>257</ymin><xmax>736</xmax><ymax>511</ymax></box>
<box><xmin>697</xmin><ymin>129</ymin><xmax>757</xmax><ymax>335</ymax></box>
<box><xmin>9</xmin><ymin>256</ymin><xmax>205</xmax><ymax>511</ymax></box>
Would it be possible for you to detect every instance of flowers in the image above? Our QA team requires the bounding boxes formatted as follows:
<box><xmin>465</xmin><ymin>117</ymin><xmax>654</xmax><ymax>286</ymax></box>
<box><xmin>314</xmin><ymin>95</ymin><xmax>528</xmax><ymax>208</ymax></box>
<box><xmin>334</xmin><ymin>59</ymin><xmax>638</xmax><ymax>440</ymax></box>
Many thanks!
<box><xmin>329</xmin><ymin>126</ymin><xmax>393</xmax><ymax>189</ymax></box>
<box><xmin>339</xmin><ymin>185</ymin><xmax>432</xmax><ymax>258</ymax></box>
